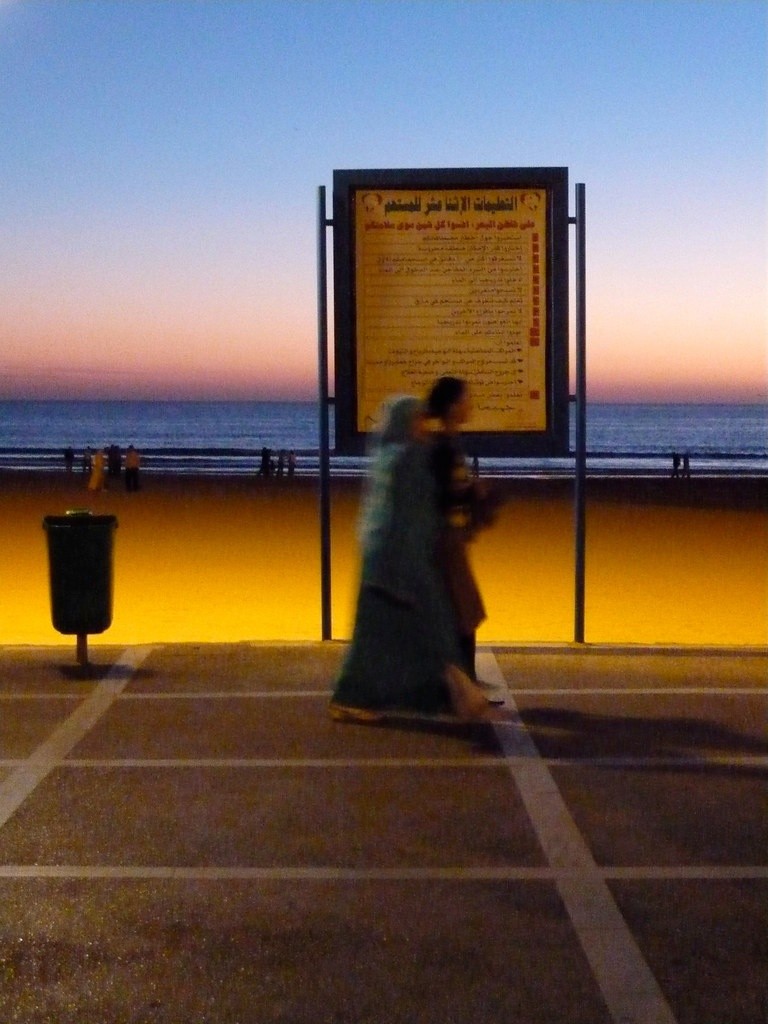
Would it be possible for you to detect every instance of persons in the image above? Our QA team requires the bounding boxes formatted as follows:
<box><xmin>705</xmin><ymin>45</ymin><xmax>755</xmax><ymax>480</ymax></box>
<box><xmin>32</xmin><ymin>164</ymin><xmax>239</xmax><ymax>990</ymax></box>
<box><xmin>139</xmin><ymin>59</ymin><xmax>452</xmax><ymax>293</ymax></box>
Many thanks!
<box><xmin>472</xmin><ymin>456</ymin><xmax>479</xmax><ymax>478</ymax></box>
<box><xmin>682</xmin><ymin>456</ymin><xmax>690</xmax><ymax>478</ymax></box>
<box><xmin>423</xmin><ymin>377</ymin><xmax>487</xmax><ymax>683</ymax></box>
<box><xmin>256</xmin><ymin>447</ymin><xmax>297</xmax><ymax>478</ymax></box>
<box><xmin>672</xmin><ymin>454</ymin><xmax>680</xmax><ymax>478</ymax></box>
<box><xmin>64</xmin><ymin>444</ymin><xmax>140</xmax><ymax>495</ymax></box>
<box><xmin>328</xmin><ymin>396</ymin><xmax>490</xmax><ymax>721</ymax></box>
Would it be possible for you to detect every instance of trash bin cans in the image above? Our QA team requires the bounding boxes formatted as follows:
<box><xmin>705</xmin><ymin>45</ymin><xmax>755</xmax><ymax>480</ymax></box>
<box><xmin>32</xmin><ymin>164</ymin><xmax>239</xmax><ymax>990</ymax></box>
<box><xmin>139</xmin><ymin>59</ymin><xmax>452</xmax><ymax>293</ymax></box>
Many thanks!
<box><xmin>43</xmin><ymin>509</ymin><xmax>119</xmax><ymax>665</ymax></box>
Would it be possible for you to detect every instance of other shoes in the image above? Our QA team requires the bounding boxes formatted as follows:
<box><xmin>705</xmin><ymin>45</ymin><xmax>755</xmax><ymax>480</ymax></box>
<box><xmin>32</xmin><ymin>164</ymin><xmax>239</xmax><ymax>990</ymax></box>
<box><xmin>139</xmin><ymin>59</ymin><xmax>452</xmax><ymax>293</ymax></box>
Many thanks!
<box><xmin>485</xmin><ymin>697</ymin><xmax>504</xmax><ymax>708</ymax></box>
<box><xmin>331</xmin><ymin>701</ymin><xmax>379</xmax><ymax>721</ymax></box>
